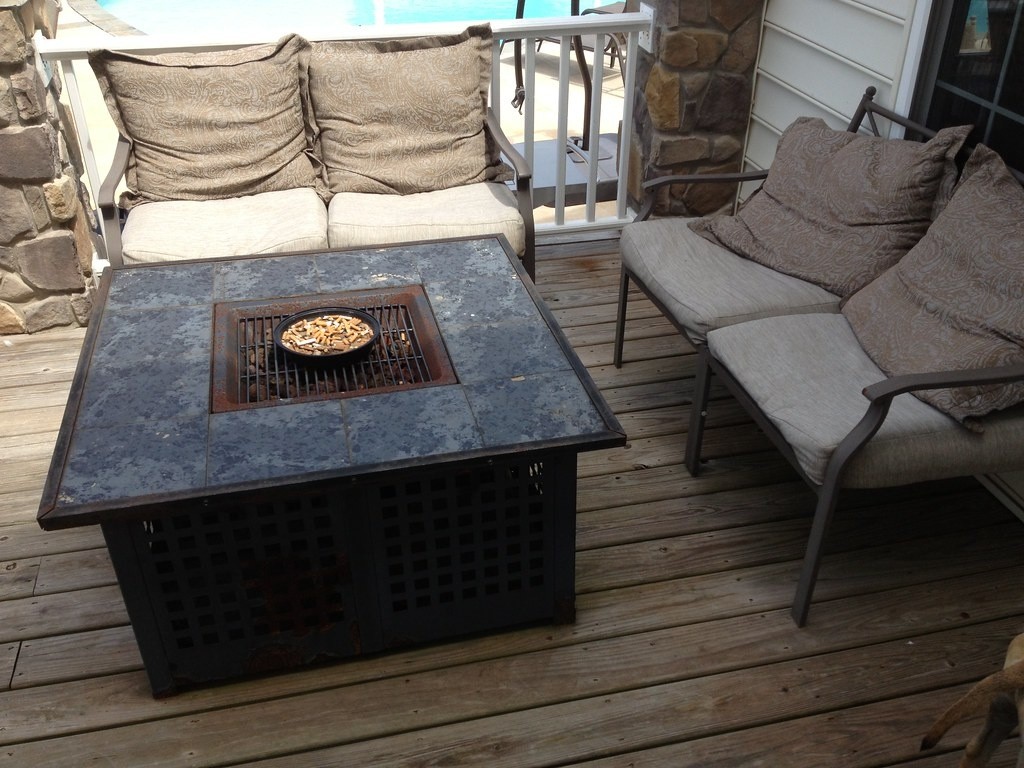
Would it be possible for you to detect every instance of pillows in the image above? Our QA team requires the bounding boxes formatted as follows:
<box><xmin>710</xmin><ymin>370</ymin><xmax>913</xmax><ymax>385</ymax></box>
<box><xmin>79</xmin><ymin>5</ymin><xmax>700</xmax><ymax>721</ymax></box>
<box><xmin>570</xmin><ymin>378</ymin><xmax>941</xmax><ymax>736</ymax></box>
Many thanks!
<box><xmin>840</xmin><ymin>141</ymin><xmax>1024</xmax><ymax>437</ymax></box>
<box><xmin>683</xmin><ymin>116</ymin><xmax>974</xmax><ymax>296</ymax></box>
<box><xmin>309</xmin><ymin>22</ymin><xmax>515</xmax><ymax>196</ymax></box>
<box><xmin>88</xmin><ymin>33</ymin><xmax>334</xmax><ymax>214</ymax></box>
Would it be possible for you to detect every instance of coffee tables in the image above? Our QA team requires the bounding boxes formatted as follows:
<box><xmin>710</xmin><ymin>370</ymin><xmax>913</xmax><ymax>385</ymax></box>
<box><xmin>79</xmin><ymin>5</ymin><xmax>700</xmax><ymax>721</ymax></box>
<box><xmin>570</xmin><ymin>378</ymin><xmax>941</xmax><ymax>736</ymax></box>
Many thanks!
<box><xmin>37</xmin><ymin>232</ymin><xmax>630</xmax><ymax>702</ymax></box>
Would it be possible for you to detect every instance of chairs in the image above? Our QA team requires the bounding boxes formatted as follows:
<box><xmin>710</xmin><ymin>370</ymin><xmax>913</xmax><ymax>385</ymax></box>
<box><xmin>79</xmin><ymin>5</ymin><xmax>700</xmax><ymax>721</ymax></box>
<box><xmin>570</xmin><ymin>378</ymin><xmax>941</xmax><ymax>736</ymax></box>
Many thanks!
<box><xmin>97</xmin><ymin>106</ymin><xmax>537</xmax><ymax>285</ymax></box>
<box><xmin>613</xmin><ymin>87</ymin><xmax>1024</xmax><ymax>629</ymax></box>
<box><xmin>500</xmin><ymin>0</ymin><xmax>641</xmax><ymax>86</ymax></box>
